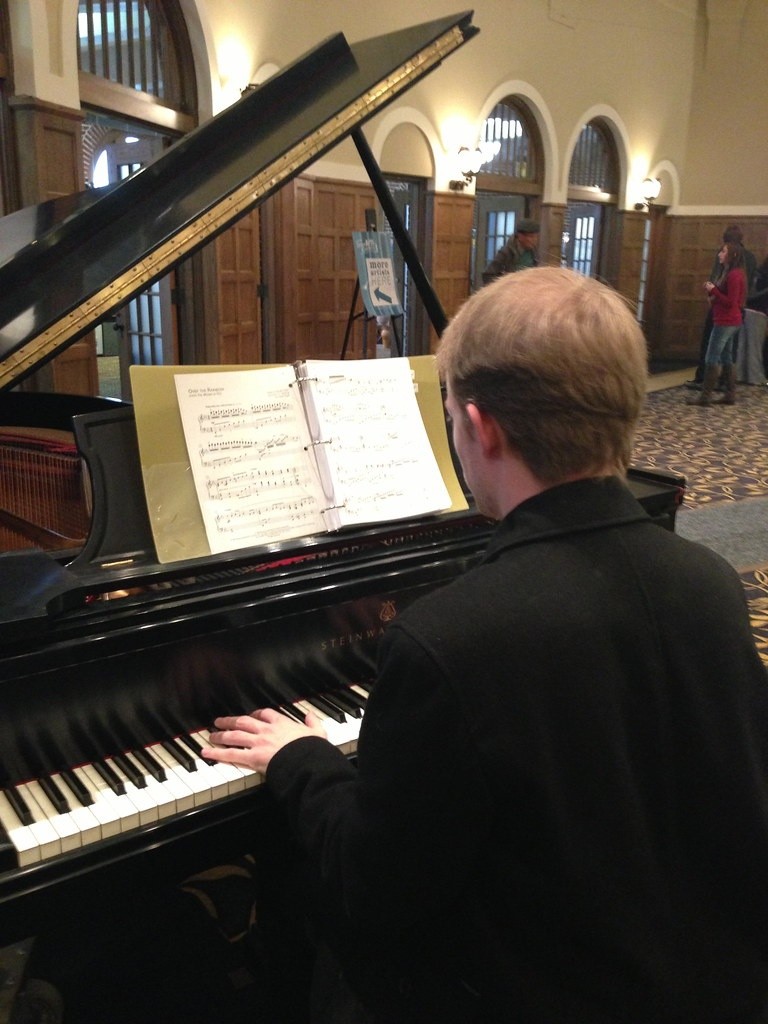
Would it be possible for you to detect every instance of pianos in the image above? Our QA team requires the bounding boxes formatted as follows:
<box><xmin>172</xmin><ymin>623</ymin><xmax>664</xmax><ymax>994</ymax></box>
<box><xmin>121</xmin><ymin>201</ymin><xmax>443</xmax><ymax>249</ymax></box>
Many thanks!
<box><xmin>1</xmin><ymin>8</ymin><xmax>691</xmax><ymax>944</ymax></box>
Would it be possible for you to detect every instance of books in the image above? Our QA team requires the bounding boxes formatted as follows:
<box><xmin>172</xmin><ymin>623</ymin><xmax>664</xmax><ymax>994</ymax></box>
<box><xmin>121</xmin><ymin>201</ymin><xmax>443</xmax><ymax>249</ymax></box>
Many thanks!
<box><xmin>128</xmin><ymin>351</ymin><xmax>470</xmax><ymax>564</ymax></box>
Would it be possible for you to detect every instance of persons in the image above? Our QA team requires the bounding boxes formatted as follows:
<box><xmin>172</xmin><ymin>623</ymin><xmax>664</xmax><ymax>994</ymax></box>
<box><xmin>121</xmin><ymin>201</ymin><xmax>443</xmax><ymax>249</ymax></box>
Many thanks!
<box><xmin>481</xmin><ymin>218</ymin><xmax>540</xmax><ymax>284</ymax></box>
<box><xmin>199</xmin><ymin>260</ymin><xmax>768</xmax><ymax>1024</ymax></box>
<box><xmin>684</xmin><ymin>225</ymin><xmax>768</xmax><ymax>406</ymax></box>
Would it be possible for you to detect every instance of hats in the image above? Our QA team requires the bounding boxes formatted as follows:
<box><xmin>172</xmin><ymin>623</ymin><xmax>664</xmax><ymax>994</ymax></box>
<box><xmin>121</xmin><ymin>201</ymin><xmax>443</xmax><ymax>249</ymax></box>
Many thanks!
<box><xmin>517</xmin><ymin>218</ymin><xmax>540</xmax><ymax>233</ymax></box>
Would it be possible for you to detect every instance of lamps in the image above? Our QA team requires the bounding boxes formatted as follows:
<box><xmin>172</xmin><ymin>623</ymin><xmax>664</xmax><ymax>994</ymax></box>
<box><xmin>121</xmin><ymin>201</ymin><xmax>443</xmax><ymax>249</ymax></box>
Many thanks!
<box><xmin>450</xmin><ymin>147</ymin><xmax>482</xmax><ymax>191</ymax></box>
<box><xmin>635</xmin><ymin>177</ymin><xmax>661</xmax><ymax>211</ymax></box>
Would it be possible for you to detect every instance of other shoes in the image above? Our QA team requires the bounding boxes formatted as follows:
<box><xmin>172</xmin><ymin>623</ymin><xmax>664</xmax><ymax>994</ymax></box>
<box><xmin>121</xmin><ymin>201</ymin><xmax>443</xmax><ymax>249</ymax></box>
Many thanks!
<box><xmin>685</xmin><ymin>380</ymin><xmax>704</xmax><ymax>390</ymax></box>
<box><xmin>713</xmin><ymin>379</ymin><xmax>728</xmax><ymax>391</ymax></box>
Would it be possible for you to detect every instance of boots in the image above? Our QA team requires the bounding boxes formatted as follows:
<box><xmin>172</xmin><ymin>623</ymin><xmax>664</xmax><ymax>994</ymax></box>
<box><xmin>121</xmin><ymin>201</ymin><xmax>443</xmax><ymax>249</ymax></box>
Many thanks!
<box><xmin>712</xmin><ymin>364</ymin><xmax>736</xmax><ymax>405</ymax></box>
<box><xmin>687</xmin><ymin>368</ymin><xmax>716</xmax><ymax>406</ymax></box>
